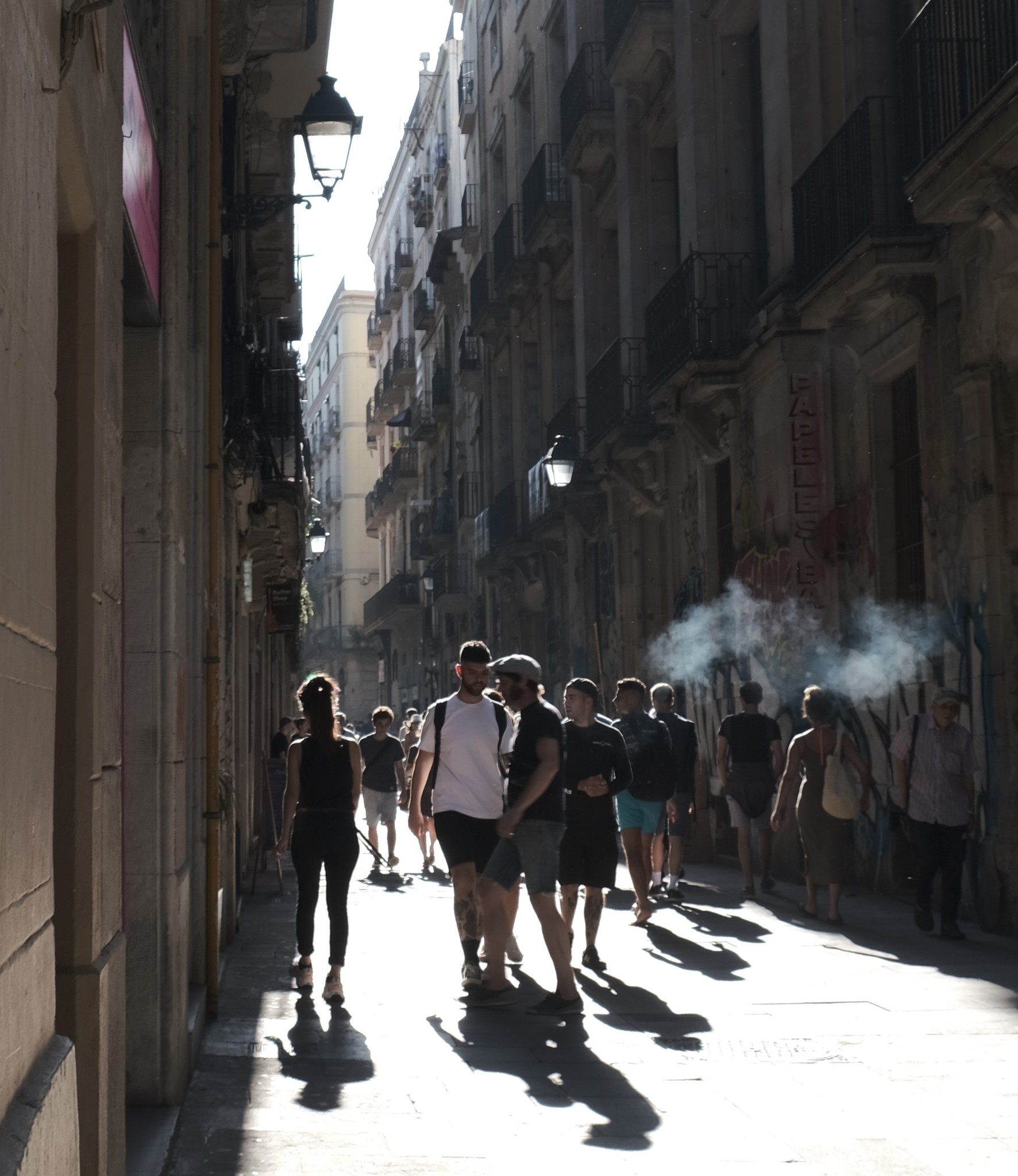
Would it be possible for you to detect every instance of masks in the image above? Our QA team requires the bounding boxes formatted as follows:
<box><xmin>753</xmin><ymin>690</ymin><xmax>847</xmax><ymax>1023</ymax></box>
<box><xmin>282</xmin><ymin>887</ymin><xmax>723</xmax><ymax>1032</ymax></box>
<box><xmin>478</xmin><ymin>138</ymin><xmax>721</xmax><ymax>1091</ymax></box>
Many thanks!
<box><xmin>411</xmin><ymin>722</ymin><xmax>422</xmax><ymax>730</ymax></box>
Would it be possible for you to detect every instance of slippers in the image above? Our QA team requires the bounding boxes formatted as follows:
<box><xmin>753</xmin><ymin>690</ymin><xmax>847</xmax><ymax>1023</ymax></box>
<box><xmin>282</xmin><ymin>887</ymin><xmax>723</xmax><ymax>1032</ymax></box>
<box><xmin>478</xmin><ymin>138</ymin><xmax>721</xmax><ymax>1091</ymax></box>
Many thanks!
<box><xmin>629</xmin><ymin>910</ymin><xmax>655</xmax><ymax>929</ymax></box>
<box><xmin>798</xmin><ymin>902</ymin><xmax>817</xmax><ymax>918</ymax></box>
<box><xmin>828</xmin><ymin>914</ymin><xmax>844</xmax><ymax>926</ymax></box>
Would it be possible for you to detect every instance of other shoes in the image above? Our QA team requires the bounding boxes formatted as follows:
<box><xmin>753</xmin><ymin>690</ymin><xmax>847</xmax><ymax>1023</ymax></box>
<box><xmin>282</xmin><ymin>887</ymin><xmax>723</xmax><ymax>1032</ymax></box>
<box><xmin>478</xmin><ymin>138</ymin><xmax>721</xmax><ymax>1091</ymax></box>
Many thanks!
<box><xmin>666</xmin><ymin>888</ymin><xmax>686</xmax><ymax>902</ymax></box>
<box><xmin>940</xmin><ymin>925</ymin><xmax>967</xmax><ymax>940</ymax></box>
<box><xmin>648</xmin><ymin>882</ymin><xmax>665</xmax><ymax>897</ymax></box>
<box><xmin>760</xmin><ymin>875</ymin><xmax>775</xmax><ymax>892</ymax></box>
<box><xmin>738</xmin><ymin>886</ymin><xmax>756</xmax><ymax>899</ymax></box>
<box><xmin>387</xmin><ymin>854</ymin><xmax>399</xmax><ymax>867</ymax></box>
<box><xmin>423</xmin><ymin>851</ymin><xmax>434</xmax><ymax>867</ymax></box>
<box><xmin>913</xmin><ymin>898</ymin><xmax>935</xmax><ymax>931</ymax></box>
<box><xmin>373</xmin><ymin>858</ymin><xmax>381</xmax><ymax>868</ymax></box>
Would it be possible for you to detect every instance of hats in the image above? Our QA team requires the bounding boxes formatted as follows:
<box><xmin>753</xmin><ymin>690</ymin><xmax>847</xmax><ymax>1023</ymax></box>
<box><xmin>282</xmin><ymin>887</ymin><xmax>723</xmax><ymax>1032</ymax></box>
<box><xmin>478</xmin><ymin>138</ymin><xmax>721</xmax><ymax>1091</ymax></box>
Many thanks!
<box><xmin>486</xmin><ymin>653</ymin><xmax>543</xmax><ymax>683</ymax></box>
<box><xmin>567</xmin><ymin>677</ymin><xmax>605</xmax><ymax>714</ymax></box>
<box><xmin>278</xmin><ymin>717</ymin><xmax>292</xmax><ymax>730</ymax></box>
<box><xmin>932</xmin><ymin>686</ymin><xmax>961</xmax><ymax>707</ymax></box>
<box><xmin>740</xmin><ymin>681</ymin><xmax>764</xmax><ymax>702</ymax></box>
<box><xmin>402</xmin><ymin>714</ymin><xmax>425</xmax><ymax>730</ymax></box>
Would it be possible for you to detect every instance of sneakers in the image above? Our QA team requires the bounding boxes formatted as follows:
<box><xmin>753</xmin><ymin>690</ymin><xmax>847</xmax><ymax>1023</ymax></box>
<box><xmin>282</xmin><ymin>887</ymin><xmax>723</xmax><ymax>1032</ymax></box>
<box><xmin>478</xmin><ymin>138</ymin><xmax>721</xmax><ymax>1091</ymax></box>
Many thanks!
<box><xmin>526</xmin><ymin>989</ymin><xmax>584</xmax><ymax>1015</ymax></box>
<box><xmin>457</xmin><ymin>978</ymin><xmax>517</xmax><ymax>1008</ymax></box>
<box><xmin>477</xmin><ymin>943</ymin><xmax>490</xmax><ymax>962</ymax></box>
<box><xmin>296</xmin><ymin>957</ymin><xmax>314</xmax><ymax>988</ymax></box>
<box><xmin>582</xmin><ymin>945</ymin><xmax>605</xmax><ymax>971</ymax></box>
<box><xmin>507</xmin><ymin>933</ymin><xmax>523</xmax><ymax>962</ymax></box>
<box><xmin>322</xmin><ymin>971</ymin><xmax>344</xmax><ymax>1002</ymax></box>
<box><xmin>459</xmin><ymin>956</ymin><xmax>483</xmax><ymax>989</ymax></box>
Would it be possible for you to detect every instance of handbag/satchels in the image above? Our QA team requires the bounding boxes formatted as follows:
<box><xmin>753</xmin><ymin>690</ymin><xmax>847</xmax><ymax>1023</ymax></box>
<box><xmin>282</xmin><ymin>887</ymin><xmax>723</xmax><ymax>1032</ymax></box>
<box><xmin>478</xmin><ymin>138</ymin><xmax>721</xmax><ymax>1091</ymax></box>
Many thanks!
<box><xmin>821</xmin><ymin>720</ymin><xmax>863</xmax><ymax>819</ymax></box>
<box><xmin>885</xmin><ymin>788</ymin><xmax>917</xmax><ymax>841</ymax></box>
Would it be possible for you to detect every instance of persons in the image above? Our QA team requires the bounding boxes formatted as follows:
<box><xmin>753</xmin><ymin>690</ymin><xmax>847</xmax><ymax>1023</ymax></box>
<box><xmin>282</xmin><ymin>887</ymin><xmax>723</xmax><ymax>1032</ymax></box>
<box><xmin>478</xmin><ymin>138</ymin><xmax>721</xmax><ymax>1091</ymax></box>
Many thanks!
<box><xmin>272</xmin><ymin>641</ymin><xmax>983</xmax><ymax>1015</ymax></box>
